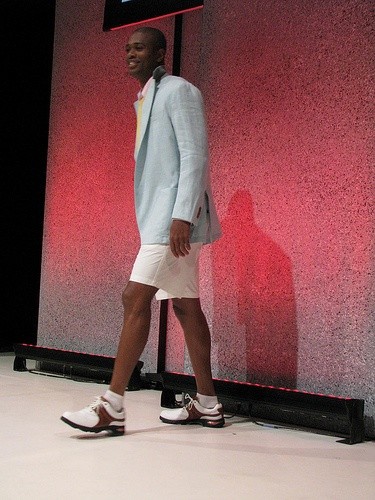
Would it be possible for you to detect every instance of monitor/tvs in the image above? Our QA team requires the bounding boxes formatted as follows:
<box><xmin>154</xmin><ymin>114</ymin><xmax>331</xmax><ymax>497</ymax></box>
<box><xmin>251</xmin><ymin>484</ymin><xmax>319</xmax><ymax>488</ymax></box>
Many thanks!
<box><xmin>102</xmin><ymin>0</ymin><xmax>204</xmax><ymax>33</ymax></box>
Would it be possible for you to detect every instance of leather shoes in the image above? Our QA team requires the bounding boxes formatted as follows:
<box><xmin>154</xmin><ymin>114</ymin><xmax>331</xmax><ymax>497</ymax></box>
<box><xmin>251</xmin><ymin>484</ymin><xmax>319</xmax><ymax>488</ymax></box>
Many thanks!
<box><xmin>160</xmin><ymin>395</ymin><xmax>225</xmax><ymax>428</ymax></box>
<box><xmin>61</xmin><ymin>396</ymin><xmax>125</xmax><ymax>436</ymax></box>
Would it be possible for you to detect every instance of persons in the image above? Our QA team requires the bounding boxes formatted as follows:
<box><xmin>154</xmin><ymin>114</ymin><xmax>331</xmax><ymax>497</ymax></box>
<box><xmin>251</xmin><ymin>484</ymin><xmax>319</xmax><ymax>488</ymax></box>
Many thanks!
<box><xmin>59</xmin><ymin>27</ymin><xmax>225</xmax><ymax>436</ymax></box>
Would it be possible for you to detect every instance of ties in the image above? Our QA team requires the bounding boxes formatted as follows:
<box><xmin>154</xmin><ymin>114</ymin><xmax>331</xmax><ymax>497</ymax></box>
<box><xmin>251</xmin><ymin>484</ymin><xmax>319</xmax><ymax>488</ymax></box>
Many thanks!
<box><xmin>135</xmin><ymin>98</ymin><xmax>144</xmax><ymax>149</ymax></box>
